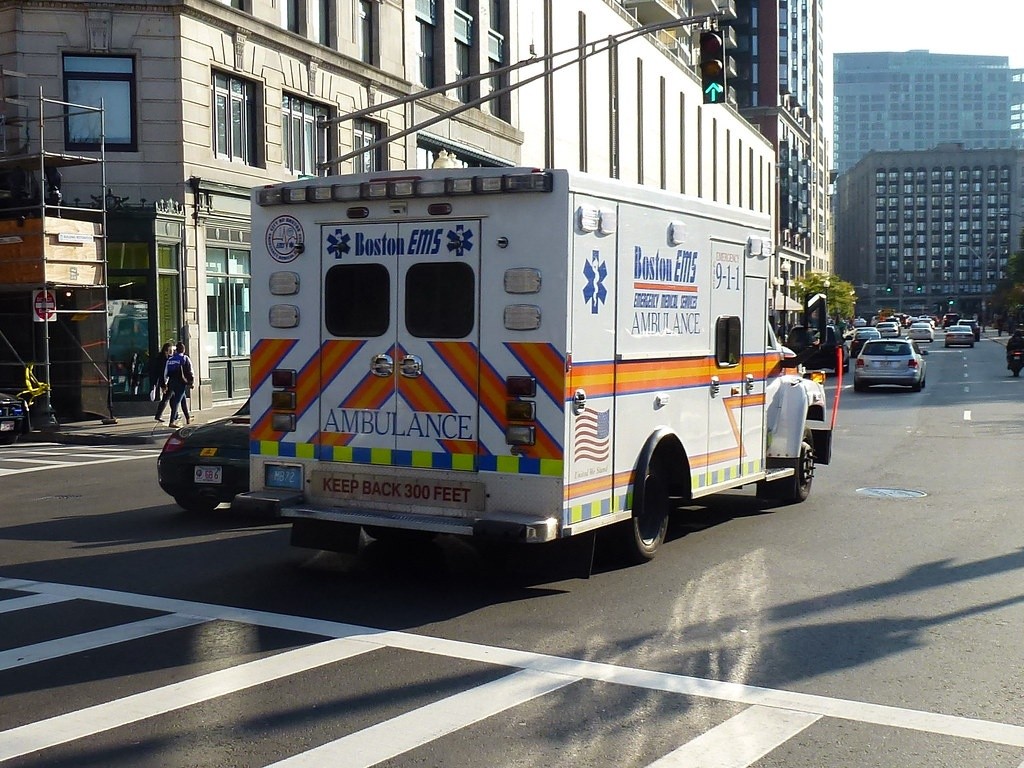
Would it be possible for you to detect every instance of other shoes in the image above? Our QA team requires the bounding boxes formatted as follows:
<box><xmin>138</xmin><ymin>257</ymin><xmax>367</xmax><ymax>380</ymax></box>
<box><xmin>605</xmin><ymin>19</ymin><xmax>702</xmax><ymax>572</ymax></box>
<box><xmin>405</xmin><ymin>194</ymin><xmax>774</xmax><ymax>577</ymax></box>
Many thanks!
<box><xmin>155</xmin><ymin>417</ymin><xmax>165</xmax><ymax>422</ymax></box>
<box><xmin>169</xmin><ymin>424</ymin><xmax>180</xmax><ymax>428</ymax></box>
<box><xmin>187</xmin><ymin>419</ymin><xmax>194</xmax><ymax>424</ymax></box>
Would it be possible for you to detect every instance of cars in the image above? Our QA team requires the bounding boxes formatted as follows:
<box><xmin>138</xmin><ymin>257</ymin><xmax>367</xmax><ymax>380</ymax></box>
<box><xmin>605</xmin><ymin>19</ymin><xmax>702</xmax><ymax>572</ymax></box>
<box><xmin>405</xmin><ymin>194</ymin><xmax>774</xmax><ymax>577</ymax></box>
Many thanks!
<box><xmin>783</xmin><ymin>323</ymin><xmax>853</xmax><ymax>373</ymax></box>
<box><xmin>853</xmin><ymin>336</ymin><xmax>929</xmax><ymax>392</ymax></box>
<box><xmin>0</xmin><ymin>394</ymin><xmax>32</xmax><ymax>445</ymax></box>
<box><xmin>156</xmin><ymin>397</ymin><xmax>250</xmax><ymax>514</ymax></box>
<box><xmin>851</xmin><ymin>331</ymin><xmax>882</xmax><ymax>357</ymax></box>
<box><xmin>854</xmin><ymin>318</ymin><xmax>867</xmax><ymax>327</ymax></box>
<box><xmin>908</xmin><ymin>323</ymin><xmax>935</xmax><ymax>342</ymax></box>
<box><xmin>852</xmin><ymin>326</ymin><xmax>877</xmax><ymax>335</ymax></box>
<box><xmin>870</xmin><ymin>315</ymin><xmax>938</xmax><ymax>329</ymax></box>
<box><xmin>943</xmin><ymin>325</ymin><xmax>977</xmax><ymax>348</ymax></box>
<box><xmin>875</xmin><ymin>322</ymin><xmax>900</xmax><ymax>338</ymax></box>
<box><xmin>956</xmin><ymin>319</ymin><xmax>981</xmax><ymax>342</ymax></box>
<box><xmin>941</xmin><ymin>314</ymin><xmax>962</xmax><ymax>330</ymax></box>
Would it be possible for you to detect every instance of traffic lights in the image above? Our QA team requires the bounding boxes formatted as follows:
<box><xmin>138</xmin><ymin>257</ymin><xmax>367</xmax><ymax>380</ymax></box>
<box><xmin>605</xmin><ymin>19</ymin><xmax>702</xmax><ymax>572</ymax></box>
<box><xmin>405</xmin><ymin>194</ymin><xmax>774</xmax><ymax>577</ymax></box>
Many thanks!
<box><xmin>699</xmin><ymin>28</ymin><xmax>726</xmax><ymax>106</ymax></box>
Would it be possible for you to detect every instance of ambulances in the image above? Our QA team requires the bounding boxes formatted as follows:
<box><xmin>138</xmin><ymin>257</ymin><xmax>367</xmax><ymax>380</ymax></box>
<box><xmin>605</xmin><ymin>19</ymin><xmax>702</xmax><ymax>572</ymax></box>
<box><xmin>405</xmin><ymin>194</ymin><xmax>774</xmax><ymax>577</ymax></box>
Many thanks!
<box><xmin>230</xmin><ymin>164</ymin><xmax>846</xmax><ymax>580</ymax></box>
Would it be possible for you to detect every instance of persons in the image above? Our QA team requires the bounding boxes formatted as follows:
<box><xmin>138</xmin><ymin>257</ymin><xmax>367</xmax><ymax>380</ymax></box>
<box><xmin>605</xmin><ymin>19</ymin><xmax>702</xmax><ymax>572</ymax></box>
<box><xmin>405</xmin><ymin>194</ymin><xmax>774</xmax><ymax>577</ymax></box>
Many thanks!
<box><xmin>775</xmin><ymin>313</ymin><xmax>856</xmax><ymax>346</ymax></box>
<box><xmin>1006</xmin><ymin>329</ymin><xmax>1024</xmax><ymax>369</ymax></box>
<box><xmin>164</xmin><ymin>344</ymin><xmax>195</xmax><ymax>427</ymax></box>
<box><xmin>998</xmin><ymin>315</ymin><xmax>1004</xmax><ymax>336</ymax></box>
<box><xmin>152</xmin><ymin>343</ymin><xmax>182</xmax><ymax>421</ymax></box>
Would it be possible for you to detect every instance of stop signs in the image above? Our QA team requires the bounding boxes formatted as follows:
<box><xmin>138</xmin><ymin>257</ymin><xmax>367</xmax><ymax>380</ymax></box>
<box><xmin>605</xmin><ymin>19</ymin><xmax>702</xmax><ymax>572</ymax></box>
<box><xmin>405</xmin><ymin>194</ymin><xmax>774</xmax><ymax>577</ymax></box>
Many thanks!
<box><xmin>35</xmin><ymin>290</ymin><xmax>55</xmax><ymax>320</ymax></box>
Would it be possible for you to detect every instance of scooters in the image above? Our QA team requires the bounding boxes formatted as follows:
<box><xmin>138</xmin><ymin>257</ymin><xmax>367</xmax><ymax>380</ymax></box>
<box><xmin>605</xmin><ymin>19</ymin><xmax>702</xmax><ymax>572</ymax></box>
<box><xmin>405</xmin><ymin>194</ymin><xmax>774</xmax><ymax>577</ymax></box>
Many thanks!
<box><xmin>1005</xmin><ymin>345</ymin><xmax>1024</xmax><ymax>377</ymax></box>
